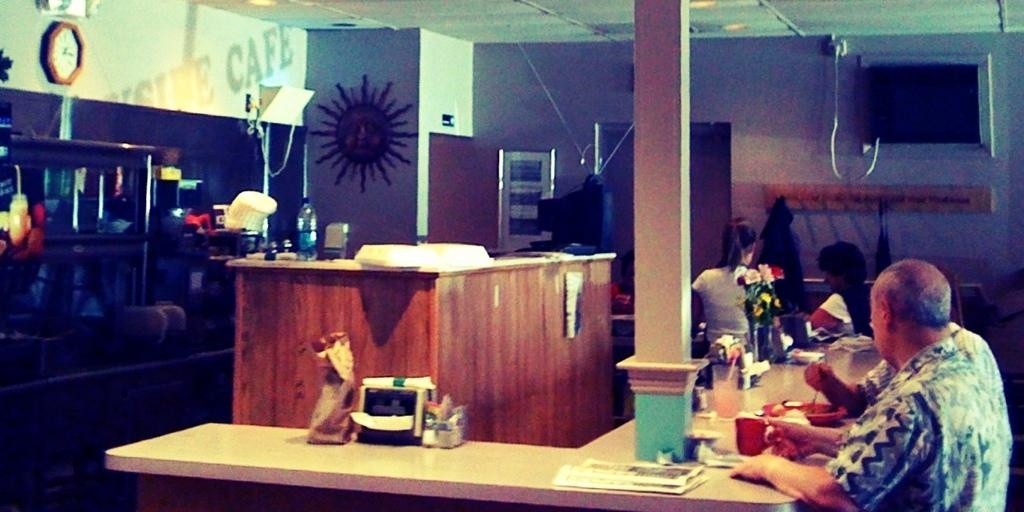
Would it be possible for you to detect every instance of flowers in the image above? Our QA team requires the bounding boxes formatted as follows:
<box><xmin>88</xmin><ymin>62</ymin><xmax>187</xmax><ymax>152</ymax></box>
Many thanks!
<box><xmin>737</xmin><ymin>260</ymin><xmax>784</xmax><ymax>324</ymax></box>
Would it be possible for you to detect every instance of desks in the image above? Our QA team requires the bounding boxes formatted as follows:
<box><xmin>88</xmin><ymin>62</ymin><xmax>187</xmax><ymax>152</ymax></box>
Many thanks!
<box><xmin>101</xmin><ymin>341</ymin><xmax>884</xmax><ymax>511</ymax></box>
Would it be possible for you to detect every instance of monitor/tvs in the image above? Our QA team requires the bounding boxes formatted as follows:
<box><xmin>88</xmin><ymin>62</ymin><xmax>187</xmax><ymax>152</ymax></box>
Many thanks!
<box><xmin>858</xmin><ymin>53</ymin><xmax>995</xmax><ymax>159</ymax></box>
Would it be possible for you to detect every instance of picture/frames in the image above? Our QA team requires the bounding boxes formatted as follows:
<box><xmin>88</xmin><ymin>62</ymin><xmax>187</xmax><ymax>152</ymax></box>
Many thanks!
<box><xmin>497</xmin><ymin>147</ymin><xmax>555</xmax><ymax>248</ymax></box>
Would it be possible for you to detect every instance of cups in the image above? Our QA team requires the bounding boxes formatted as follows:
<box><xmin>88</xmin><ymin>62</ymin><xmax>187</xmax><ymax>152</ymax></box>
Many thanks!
<box><xmin>733</xmin><ymin>416</ymin><xmax>780</xmax><ymax>457</ymax></box>
<box><xmin>710</xmin><ymin>364</ymin><xmax>739</xmax><ymax>419</ymax></box>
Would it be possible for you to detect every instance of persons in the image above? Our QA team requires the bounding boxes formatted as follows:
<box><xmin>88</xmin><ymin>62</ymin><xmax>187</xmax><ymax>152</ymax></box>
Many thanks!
<box><xmin>804</xmin><ymin>321</ymin><xmax>1012</xmax><ymax>462</ymax></box>
<box><xmin>611</xmin><ymin>249</ymin><xmax>634</xmax><ymax>315</ymax></box>
<box><xmin>793</xmin><ymin>242</ymin><xmax>876</xmax><ymax>344</ymax></box>
<box><xmin>729</xmin><ymin>260</ymin><xmax>1014</xmax><ymax>512</ymax></box>
<box><xmin>690</xmin><ymin>218</ymin><xmax>759</xmax><ymax>353</ymax></box>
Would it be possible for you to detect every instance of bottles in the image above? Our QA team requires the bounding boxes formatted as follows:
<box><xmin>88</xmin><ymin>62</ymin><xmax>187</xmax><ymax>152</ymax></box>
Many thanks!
<box><xmin>295</xmin><ymin>198</ymin><xmax>316</xmax><ymax>262</ymax></box>
<box><xmin>8</xmin><ymin>192</ymin><xmax>27</xmax><ymax>247</ymax></box>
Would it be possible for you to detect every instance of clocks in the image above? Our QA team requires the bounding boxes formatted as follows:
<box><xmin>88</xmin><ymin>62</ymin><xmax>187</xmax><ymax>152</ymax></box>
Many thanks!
<box><xmin>38</xmin><ymin>21</ymin><xmax>85</xmax><ymax>86</ymax></box>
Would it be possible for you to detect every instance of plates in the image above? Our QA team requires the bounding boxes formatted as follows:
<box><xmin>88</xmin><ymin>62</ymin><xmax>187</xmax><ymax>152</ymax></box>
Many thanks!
<box><xmin>761</xmin><ymin>400</ymin><xmax>848</xmax><ymax>424</ymax></box>
